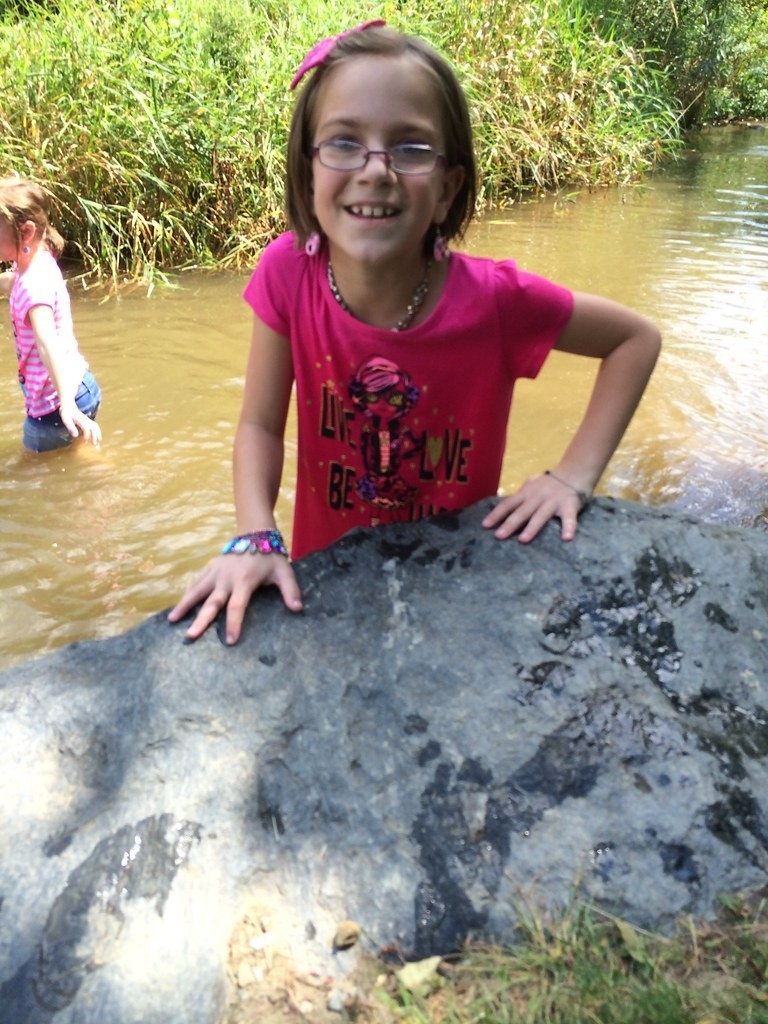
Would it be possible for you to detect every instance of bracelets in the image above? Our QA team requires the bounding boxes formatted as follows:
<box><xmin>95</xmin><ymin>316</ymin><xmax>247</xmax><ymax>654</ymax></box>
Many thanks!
<box><xmin>221</xmin><ymin>529</ymin><xmax>288</xmax><ymax>555</ymax></box>
<box><xmin>545</xmin><ymin>470</ymin><xmax>587</xmax><ymax>504</ymax></box>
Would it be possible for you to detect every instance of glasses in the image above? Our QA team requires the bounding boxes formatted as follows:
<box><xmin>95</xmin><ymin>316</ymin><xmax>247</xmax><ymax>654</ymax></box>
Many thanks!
<box><xmin>307</xmin><ymin>138</ymin><xmax>455</xmax><ymax>177</ymax></box>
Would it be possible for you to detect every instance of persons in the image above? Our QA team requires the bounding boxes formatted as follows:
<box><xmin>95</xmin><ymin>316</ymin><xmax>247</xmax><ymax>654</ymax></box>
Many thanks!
<box><xmin>167</xmin><ymin>20</ymin><xmax>662</xmax><ymax>647</ymax></box>
<box><xmin>0</xmin><ymin>177</ymin><xmax>102</xmax><ymax>453</ymax></box>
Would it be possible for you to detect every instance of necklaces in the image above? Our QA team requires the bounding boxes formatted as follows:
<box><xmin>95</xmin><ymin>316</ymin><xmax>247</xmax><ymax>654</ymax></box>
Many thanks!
<box><xmin>327</xmin><ymin>258</ymin><xmax>432</xmax><ymax>333</ymax></box>
